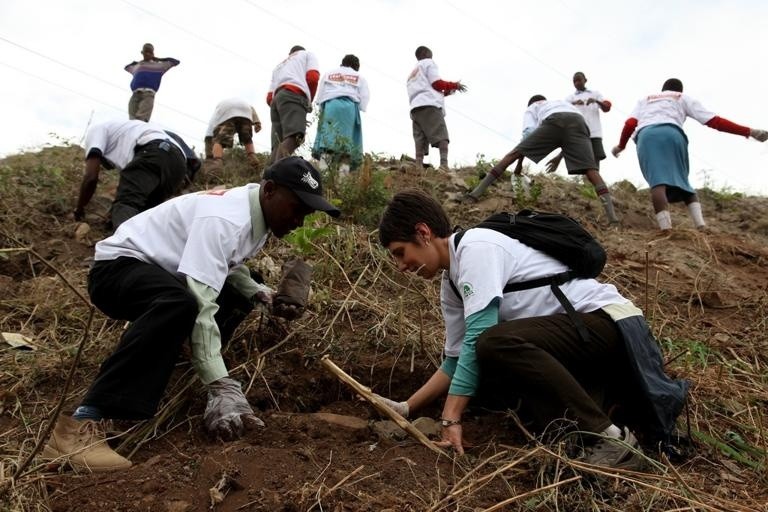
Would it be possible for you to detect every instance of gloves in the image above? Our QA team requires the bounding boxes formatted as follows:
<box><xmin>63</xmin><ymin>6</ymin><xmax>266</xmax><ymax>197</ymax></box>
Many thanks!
<box><xmin>355</xmin><ymin>391</ymin><xmax>410</xmax><ymax>429</ymax></box>
<box><xmin>252</xmin><ymin>286</ymin><xmax>307</xmax><ymax>320</ymax></box>
<box><xmin>752</xmin><ymin>129</ymin><xmax>768</xmax><ymax>145</ymax></box>
<box><xmin>202</xmin><ymin>379</ymin><xmax>266</xmax><ymax>444</ymax></box>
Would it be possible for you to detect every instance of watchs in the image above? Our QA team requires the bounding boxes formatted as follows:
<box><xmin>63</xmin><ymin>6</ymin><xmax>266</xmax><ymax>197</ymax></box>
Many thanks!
<box><xmin>439</xmin><ymin>419</ymin><xmax>461</xmax><ymax>427</ymax></box>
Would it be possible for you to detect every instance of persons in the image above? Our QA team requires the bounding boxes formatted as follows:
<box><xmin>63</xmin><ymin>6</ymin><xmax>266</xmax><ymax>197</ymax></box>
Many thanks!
<box><xmin>71</xmin><ymin>120</ymin><xmax>201</xmax><ymax>237</ymax></box>
<box><xmin>309</xmin><ymin>55</ymin><xmax>371</xmax><ymax>189</ymax></box>
<box><xmin>406</xmin><ymin>46</ymin><xmax>467</xmax><ymax>176</ymax></box>
<box><xmin>205</xmin><ymin>99</ymin><xmax>261</xmax><ymax>172</ymax></box>
<box><xmin>37</xmin><ymin>156</ymin><xmax>342</xmax><ymax>475</ymax></box>
<box><xmin>612</xmin><ymin>77</ymin><xmax>768</xmax><ymax>235</ymax></box>
<box><xmin>122</xmin><ymin>43</ymin><xmax>180</xmax><ymax>123</ymax></box>
<box><xmin>468</xmin><ymin>93</ymin><xmax>623</xmax><ymax>226</ymax></box>
<box><xmin>266</xmin><ymin>46</ymin><xmax>319</xmax><ymax>164</ymax></box>
<box><xmin>362</xmin><ymin>192</ymin><xmax>683</xmax><ymax>486</ymax></box>
<box><xmin>564</xmin><ymin>72</ymin><xmax>612</xmax><ymax>187</ymax></box>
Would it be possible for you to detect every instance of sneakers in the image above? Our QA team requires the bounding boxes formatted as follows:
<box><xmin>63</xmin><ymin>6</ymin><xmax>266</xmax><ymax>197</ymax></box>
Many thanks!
<box><xmin>569</xmin><ymin>426</ymin><xmax>647</xmax><ymax>492</ymax></box>
<box><xmin>39</xmin><ymin>408</ymin><xmax>132</xmax><ymax>478</ymax></box>
<box><xmin>660</xmin><ymin>228</ymin><xmax>674</xmax><ymax>239</ymax></box>
<box><xmin>697</xmin><ymin>223</ymin><xmax>714</xmax><ymax>238</ymax></box>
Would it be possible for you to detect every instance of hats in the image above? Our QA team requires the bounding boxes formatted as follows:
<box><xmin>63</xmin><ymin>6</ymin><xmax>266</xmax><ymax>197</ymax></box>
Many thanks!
<box><xmin>262</xmin><ymin>155</ymin><xmax>342</xmax><ymax>219</ymax></box>
